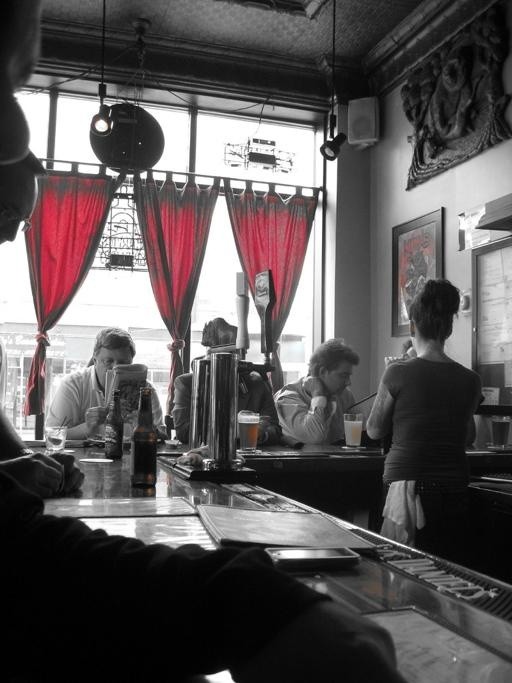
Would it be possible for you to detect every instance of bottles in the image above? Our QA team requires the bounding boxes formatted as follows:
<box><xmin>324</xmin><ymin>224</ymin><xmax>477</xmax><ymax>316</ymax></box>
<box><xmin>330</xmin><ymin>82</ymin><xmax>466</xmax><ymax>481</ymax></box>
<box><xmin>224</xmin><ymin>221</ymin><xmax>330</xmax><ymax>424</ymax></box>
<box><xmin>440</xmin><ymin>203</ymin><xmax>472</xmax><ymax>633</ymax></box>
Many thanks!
<box><xmin>105</xmin><ymin>388</ymin><xmax>124</xmax><ymax>459</ymax></box>
<box><xmin>207</xmin><ymin>351</ymin><xmax>238</xmax><ymax>463</ymax></box>
<box><xmin>188</xmin><ymin>358</ymin><xmax>209</xmax><ymax>450</ymax></box>
<box><xmin>129</xmin><ymin>385</ymin><xmax>159</xmax><ymax>489</ymax></box>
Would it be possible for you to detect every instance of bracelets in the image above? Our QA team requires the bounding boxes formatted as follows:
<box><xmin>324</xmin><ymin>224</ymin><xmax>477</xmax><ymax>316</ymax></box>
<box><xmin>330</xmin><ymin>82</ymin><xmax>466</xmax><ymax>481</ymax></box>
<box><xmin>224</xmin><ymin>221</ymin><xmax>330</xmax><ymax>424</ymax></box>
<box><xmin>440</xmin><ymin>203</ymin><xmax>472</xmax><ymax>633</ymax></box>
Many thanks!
<box><xmin>17</xmin><ymin>448</ymin><xmax>34</xmax><ymax>456</ymax></box>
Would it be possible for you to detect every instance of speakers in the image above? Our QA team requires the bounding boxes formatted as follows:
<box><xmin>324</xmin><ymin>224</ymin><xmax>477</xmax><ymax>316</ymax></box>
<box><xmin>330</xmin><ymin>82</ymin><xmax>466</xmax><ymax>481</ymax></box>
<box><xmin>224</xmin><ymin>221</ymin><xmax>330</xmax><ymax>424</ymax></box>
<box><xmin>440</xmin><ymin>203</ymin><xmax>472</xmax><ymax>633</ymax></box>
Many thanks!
<box><xmin>348</xmin><ymin>97</ymin><xmax>379</xmax><ymax>146</ymax></box>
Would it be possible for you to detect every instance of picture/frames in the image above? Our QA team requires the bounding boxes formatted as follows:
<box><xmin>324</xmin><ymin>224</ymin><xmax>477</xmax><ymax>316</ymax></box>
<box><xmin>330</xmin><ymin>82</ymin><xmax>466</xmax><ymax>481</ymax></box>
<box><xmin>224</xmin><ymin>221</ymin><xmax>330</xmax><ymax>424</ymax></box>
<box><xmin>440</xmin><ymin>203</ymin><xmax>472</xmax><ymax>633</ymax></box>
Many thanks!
<box><xmin>390</xmin><ymin>206</ymin><xmax>444</xmax><ymax>337</ymax></box>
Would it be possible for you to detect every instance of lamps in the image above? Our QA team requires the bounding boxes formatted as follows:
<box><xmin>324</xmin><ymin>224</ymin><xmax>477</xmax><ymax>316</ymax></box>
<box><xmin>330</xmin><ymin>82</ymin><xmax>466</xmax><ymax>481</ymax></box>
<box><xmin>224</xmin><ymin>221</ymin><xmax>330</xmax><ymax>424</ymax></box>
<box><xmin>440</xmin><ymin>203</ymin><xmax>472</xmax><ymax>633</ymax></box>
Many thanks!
<box><xmin>91</xmin><ymin>0</ymin><xmax>113</xmax><ymax>138</ymax></box>
<box><xmin>321</xmin><ymin>0</ymin><xmax>347</xmax><ymax>160</ymax></box>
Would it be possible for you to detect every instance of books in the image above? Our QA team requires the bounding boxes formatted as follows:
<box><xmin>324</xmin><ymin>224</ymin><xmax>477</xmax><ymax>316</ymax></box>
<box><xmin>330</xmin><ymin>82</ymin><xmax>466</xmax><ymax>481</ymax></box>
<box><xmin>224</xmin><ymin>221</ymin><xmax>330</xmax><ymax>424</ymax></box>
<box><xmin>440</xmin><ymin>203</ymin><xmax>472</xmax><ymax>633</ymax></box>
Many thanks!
<box><xmin>105</xmin><ymin>362</ymin><xmax>148</xmax><ymax>425</ymax></box>
<box><xmin>194</xmin><ymin>501</ymin><xmax>378</xmax><ymax>551</ymax></box>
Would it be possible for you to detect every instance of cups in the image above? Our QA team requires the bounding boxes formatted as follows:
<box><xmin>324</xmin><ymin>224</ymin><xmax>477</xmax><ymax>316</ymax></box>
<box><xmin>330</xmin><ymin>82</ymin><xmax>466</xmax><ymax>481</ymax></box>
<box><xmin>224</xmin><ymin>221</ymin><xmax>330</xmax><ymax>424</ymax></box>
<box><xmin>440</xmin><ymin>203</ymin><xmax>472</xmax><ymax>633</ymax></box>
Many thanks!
<box><xmin>237</xmin><ymin>412</ymin><xmax>260</xmax><ymax>452</ymax></box>
<box><xmin>44</xmin><ymin>425</ymin><xmax>68</xmax><ymax>451</ymax></box>
<box><xmin>164</xmin><ymin>439</ymin><xmax>180</xmax><ymax>449</ymax></box>
<box><xmin>343</xmin><ymin>413</ymin><xmax>364</xmax><ymax>447</ymax></box>
<box><xmin>491</xmin><ymin>415</ymin><xmax>510</xmax><ymax>446</ymax></box>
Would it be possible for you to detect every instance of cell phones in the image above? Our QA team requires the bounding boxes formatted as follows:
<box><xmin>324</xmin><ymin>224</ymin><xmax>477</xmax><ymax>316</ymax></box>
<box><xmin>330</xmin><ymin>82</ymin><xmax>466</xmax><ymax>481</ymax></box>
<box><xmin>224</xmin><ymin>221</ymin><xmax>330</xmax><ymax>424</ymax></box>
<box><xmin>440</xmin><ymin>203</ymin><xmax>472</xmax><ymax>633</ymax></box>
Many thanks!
<box><xmin>264</xmin><ymin>546</ymin><xmax>361</xmax><ymax>567</ymax></box>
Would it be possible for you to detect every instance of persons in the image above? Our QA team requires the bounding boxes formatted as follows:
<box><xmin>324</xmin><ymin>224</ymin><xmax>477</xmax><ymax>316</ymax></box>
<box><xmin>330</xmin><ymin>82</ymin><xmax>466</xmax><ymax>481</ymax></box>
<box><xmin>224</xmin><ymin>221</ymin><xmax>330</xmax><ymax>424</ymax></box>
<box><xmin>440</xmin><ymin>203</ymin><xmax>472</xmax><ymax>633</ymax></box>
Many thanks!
<box><xmin>274</xmin><ymin>336</ymin><xmax>361</xmax><ymax>445</ymax></box>
<box><xmin>1</xmin><ymin>148</ymin><xmax>48</xmax><ymax>245</ymax></box>
<box><xmin>2</xmin><ymin>468</ymin><xmax>408</xmax><ymax>681</ymax></box>
<box><xmin>170</xmin><ymin>353</ymin><xmax>283</xmax><ymax>445</ymax></box>
<box><xmin>1</xmin><ymin>411</ymin><xmax>86</xmax><ymax>499</ymax></box>
<box><xmin>364</xmin><ymin>276</ymin><xmax>483</xmax><ymax>553</ymax></box>
<box><xmin>0</xmin><ymin>0</ymin><xmax>45</xmax><ymax>165</ymax></box>
<box><xmin>380</xmin><ymin>339</ymin><xmax>477</xmax><ymax>446</ymax></box>
<box><xmin>44</xmin><ymin>324</ymin><xmax>167</xmax><ymax>443</ymax></box>
